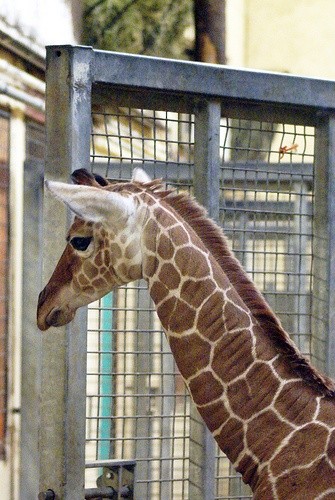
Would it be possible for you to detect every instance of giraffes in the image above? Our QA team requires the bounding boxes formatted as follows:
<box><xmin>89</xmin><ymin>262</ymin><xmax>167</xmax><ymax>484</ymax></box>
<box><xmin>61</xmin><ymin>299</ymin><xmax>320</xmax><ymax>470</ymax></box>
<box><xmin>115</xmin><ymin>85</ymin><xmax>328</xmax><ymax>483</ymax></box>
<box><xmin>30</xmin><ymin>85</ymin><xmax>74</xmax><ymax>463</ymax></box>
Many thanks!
<box><xmin>36</xmin><ymin>168</ymin><xmax>335</xmax><ymax>500</ymax></box>
